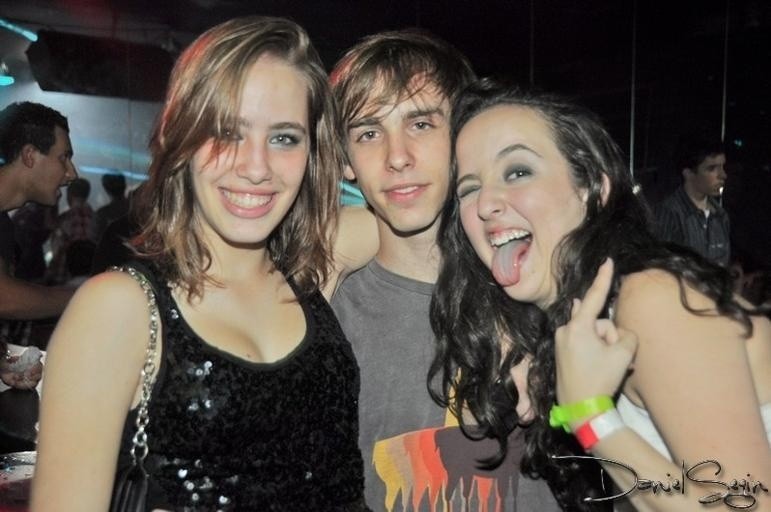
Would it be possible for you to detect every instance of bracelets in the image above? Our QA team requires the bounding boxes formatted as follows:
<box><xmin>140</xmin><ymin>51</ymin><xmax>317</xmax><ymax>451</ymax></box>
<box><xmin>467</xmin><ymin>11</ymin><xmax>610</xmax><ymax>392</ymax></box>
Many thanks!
<box><xmin>574</xmin><ymin>409</ymin><xmax>624</xmax><ymax>449</ymax></box>
<box><xmin>547</xmin><ymin>396</ymin><xmax>614</xmax><ymax>433</ymax></box>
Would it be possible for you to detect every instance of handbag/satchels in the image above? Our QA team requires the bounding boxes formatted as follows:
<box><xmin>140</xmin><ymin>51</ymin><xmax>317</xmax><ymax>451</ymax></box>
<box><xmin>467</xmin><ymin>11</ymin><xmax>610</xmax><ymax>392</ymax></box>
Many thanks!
<box><xmin>111</xmin><ymin>465</ymin><xmax>148</xmax><ymax>512</ymax></box>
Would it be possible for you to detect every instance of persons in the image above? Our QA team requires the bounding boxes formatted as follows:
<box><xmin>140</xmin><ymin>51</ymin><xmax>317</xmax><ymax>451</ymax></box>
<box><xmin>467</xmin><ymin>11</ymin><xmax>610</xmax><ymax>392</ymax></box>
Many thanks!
<box><xmin>1</xmin><ymin>100</ymin><xmax>731</xmax><ymax>393</ymax></box>
<box><xmin>429</xmin><ymin>72</ymin><xmax>771</xmax><ymax>510</ymax></box>
<box><xmin>27</xmin><ymin>13</ymin><xmax>382</xmax><ymax>512</ymax></box>
<box><xmin>328</xmin><ymin>29</ymin><xmax>565</xmax><ymax>512</ymax></box>
